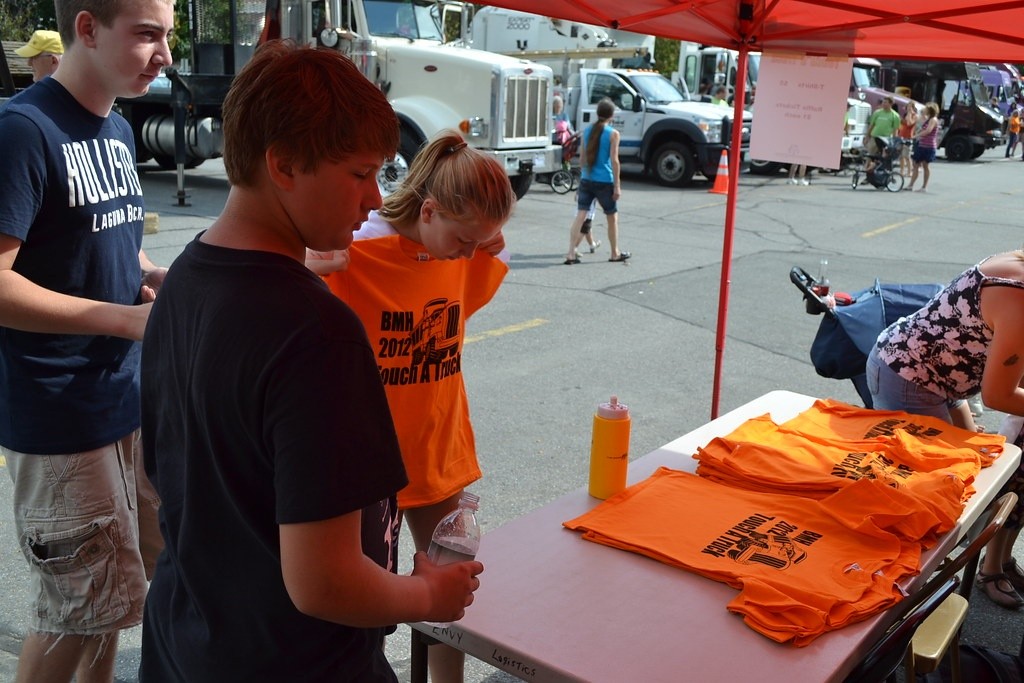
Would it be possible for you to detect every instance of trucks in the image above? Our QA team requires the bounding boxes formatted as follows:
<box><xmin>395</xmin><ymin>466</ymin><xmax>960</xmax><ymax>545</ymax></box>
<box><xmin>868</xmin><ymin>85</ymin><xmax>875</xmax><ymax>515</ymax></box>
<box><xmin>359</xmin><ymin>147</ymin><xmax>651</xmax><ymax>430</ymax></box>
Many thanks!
<box><xmin>593</xmin><ymin>28</ymin><xmax>871</xmax><ymax>168</ymax></box>
<box><xmin>435</xmin><ymin>2</ymin><xmax>757</xmax><ymax>187</ymax></box>
<box><xmin>849</xmin><ymin>60</ymin><xmax>1024</xmax><ymax>163</ymax></box>
<box><xmin>0</xmin><ymin>1</ymin><xmax>567</xmax><ymax>214</ymax></box>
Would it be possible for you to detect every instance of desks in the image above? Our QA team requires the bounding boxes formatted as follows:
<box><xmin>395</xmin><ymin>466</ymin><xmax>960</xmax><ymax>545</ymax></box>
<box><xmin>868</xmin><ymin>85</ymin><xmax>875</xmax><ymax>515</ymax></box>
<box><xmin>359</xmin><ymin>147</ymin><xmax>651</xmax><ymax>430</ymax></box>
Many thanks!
<box><xmin>405</xmin><ymin>391</ymin><xmax>1022</xmax><ymax>683</ymax></box>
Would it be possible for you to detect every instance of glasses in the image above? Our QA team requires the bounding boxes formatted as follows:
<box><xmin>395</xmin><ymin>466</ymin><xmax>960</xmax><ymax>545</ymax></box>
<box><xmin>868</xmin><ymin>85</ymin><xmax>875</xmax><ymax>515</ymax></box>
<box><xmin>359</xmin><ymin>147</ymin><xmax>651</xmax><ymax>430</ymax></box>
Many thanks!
<box><xmin>31</xmin><ymin>54</ymin><xmax>54</xmax><ymax>61</ymax></box>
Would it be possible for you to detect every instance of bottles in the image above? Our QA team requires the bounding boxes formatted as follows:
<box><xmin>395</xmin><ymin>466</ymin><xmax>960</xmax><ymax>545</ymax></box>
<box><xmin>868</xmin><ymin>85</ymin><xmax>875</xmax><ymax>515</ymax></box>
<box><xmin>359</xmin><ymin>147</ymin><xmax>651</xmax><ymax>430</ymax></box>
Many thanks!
<box><xmin>422</xmin><ymin>491</ymin><xmax>482</xmax><ymax>629</ymax></box>
<box><xmin>588</xmin><ymin>395</ymin><xmax>632</xmax><ymax>501</ymax></box>
<box><xmin>811</xmin><ymin>260</ymin><xmax>830</xmax><ymax>297</ymax></box>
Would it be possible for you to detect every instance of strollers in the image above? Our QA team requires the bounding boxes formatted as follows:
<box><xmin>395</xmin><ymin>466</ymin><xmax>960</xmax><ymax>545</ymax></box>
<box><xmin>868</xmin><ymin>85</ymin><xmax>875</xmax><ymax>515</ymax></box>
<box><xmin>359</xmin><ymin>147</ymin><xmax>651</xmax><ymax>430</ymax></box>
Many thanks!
<box><xmin>789</xmin><ymin>267</ymin><xmax>946</xmax><ymax>411</ymax></box>
<box><xmin>550</xmin><ymin>129</ymin><xmax>585</xmax><ymax>193</ymax></box>
<box><xmin>848</xmin><ymin>137</ymin><xmax>915</xmax><ymax>193</ymax></box>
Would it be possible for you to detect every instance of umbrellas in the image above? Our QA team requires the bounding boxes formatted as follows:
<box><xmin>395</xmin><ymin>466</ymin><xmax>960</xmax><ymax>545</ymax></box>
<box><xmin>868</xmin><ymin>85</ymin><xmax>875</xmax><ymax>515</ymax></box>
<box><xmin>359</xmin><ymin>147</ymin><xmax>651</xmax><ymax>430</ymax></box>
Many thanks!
<box><xmin>464</xmin><ymin>0</ymin><xmax>1023</xmax><ymax>421</ymax></box>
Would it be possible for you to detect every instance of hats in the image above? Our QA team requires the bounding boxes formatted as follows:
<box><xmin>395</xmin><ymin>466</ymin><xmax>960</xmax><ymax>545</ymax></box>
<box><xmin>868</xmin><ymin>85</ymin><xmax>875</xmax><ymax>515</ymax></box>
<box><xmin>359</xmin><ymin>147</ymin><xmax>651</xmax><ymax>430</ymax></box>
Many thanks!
<box><xmin>14</xmin><ymin>30</ymin><xmax>64</xmax><ymax>57</ymax></box>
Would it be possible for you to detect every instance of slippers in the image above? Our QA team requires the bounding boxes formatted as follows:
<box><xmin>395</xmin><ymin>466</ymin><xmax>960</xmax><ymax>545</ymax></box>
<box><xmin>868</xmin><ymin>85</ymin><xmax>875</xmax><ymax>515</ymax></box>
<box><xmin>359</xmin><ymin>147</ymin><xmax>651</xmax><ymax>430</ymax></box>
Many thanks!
<box><xmin>564</xmin><ymin>254</ymin><xmax>581</xmax><ymax>264</ymax></box>
<box><xmin>609</xmin><ymin>252</ymin><xmax>632</xmax><ymax>262</ymax></box>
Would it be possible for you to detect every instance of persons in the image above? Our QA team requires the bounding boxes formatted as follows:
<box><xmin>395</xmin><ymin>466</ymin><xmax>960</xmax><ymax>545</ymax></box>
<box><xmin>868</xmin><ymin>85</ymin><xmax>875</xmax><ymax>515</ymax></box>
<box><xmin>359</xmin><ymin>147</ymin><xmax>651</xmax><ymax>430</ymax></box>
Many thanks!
<box><xmin>786</xmin><ymin>163</ymin><xmax>811</xmax><ymax>186</ymax></box>
<box><xmin>702</xmin><ymin>87</ymin><xmax>731</xmax><ymax>107</ymax></box>
<box><xmin>0</xmin><ymin>0</ymin><xmax>175</xmax><ymax>683</ymax></box>
<box><xmin>303</xmin><ymin>127</ymin><xmax>516</xmax><ymax>683</ymax></box>
<box><xmin>989</xmin><ymin>96</ymin><xmax>1024</xmax><ymax>163</ymax></box>
<box><xmin>562</xmin><ymin>98</ymin><xmax>632</xmax><ymax>265</ymax></box>
<box><xmin>866</xmin><ymin>249</ymin><xmax>1024</xmax><ymax>618</ymax></box>
<box><xmin>552</xmin><ymin>95</ymin><xmax>576</xmax><ymax>147</ymax></box>
<box><xmin>139</xmin><ymin>40</ymin><xmax>484</xmax><ymax>683</ymax></box>
<box><xmin>842</xmin><ymin>97</ymin><xmax>940</xmax><ymax>192</ymax></box>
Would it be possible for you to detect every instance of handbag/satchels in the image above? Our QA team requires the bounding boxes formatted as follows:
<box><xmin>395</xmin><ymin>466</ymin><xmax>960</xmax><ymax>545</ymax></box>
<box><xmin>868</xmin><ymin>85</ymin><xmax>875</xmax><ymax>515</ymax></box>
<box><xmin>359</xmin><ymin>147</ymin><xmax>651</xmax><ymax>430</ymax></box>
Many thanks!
<box><xmin>556</xmin><ymin>120</ymin><xmax>571</xmax><ymax>145</ymax></box>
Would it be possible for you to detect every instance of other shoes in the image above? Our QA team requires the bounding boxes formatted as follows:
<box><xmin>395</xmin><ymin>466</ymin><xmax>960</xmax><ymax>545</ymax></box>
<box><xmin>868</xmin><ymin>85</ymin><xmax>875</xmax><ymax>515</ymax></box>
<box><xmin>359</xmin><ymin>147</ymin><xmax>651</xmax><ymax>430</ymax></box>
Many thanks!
<box><xmin>916</xmin><ymin>188</ymin><xmax>927</xmax><ymax>193</ymax></box>
<box><xmin>589</xmin><ymin>240</ymin><xmax>601</xmax><ymax>252</ymax></box>
<box><xmin>787</xmin><ymin>179</ymin><xmax>797</xmax><ymax>186</ymax></box>
<box><xmin>575</xmin><ymin>250</ymin><xmax>583</xmax><ymax>257</ymax></box>
<box><xmin>797</xmin><ymin>180</ymin><xmax>808</xmax><ymax>186</ymax></box>
<box><xmin>979</xmin><ymin>553</ymin><xmax>1024</xmax><ymax>590</ymax></box>
<box><xmin>903</xmin><ymin>186</ymin><xmax>913</xmax><ymax>191</ymax></box>
<box><xmin>976</xmin><ymin>568</ymin><xmax>1024</xmax><ymax>607</ymax></box>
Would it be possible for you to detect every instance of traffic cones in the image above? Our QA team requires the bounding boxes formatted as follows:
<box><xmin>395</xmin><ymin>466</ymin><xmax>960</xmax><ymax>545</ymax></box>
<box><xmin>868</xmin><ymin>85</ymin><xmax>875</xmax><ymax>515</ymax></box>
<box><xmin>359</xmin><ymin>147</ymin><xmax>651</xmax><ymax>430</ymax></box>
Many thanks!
<box><xmin>707</xmin><ymin>149</ymin><xmax>730</xmax><ymax>195</ymax></box>
